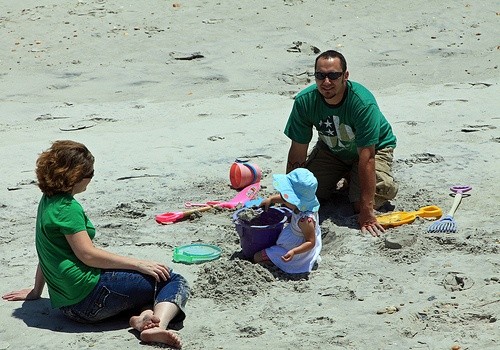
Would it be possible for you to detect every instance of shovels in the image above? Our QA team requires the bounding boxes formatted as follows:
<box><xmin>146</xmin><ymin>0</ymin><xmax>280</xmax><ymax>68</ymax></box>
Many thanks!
<box><xmin>155</xmin><ymin>181</ymin><xmax>261</xmax><ymax>225</ymax></box>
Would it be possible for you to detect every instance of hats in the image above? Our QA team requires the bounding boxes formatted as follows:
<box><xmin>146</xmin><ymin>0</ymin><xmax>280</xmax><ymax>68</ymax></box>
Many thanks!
<box><xmin>273</xmin><ymin>167</ymin><xmax>320</xmax><ymax>212</ymax></box>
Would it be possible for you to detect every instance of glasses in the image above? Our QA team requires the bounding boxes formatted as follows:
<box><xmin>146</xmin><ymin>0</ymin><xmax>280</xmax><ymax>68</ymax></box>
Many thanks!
<box><xmin>314</xmin><ymin>71</ymin><xmax>344</xmax><ymax>80</ymax></box>
<box><xmin>83</xmin><ymin>168</ymin><xmax>94</xmax><ymax>179</ymax></box>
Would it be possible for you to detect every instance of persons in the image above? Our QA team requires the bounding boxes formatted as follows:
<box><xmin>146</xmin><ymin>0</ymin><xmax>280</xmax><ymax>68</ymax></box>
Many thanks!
<box><xmin>284</xmin><ymin>50</ymin><xmax>399</xmax><ymax>238</ymax></box>
<box><xmin>2</xmin><ymin>141</ymin><xmax>189</xmax><ymax>349</ymax></box>
<box><xmin>253</xmin><ymin>168</ymin><xmax>321</xmax><ymax>273</ymax></box>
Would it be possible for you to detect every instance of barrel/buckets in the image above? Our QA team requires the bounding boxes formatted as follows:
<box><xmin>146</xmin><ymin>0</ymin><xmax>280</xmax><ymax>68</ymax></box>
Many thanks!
<box><xmin>229</xmin><ymin>162</ymin><xmax>261</xmax><ymax>190</ymax></box>
<box><xmin>233</xmin><ymin>206</ymin><xmax>293</xmax><ymax>259</ymax></box>
